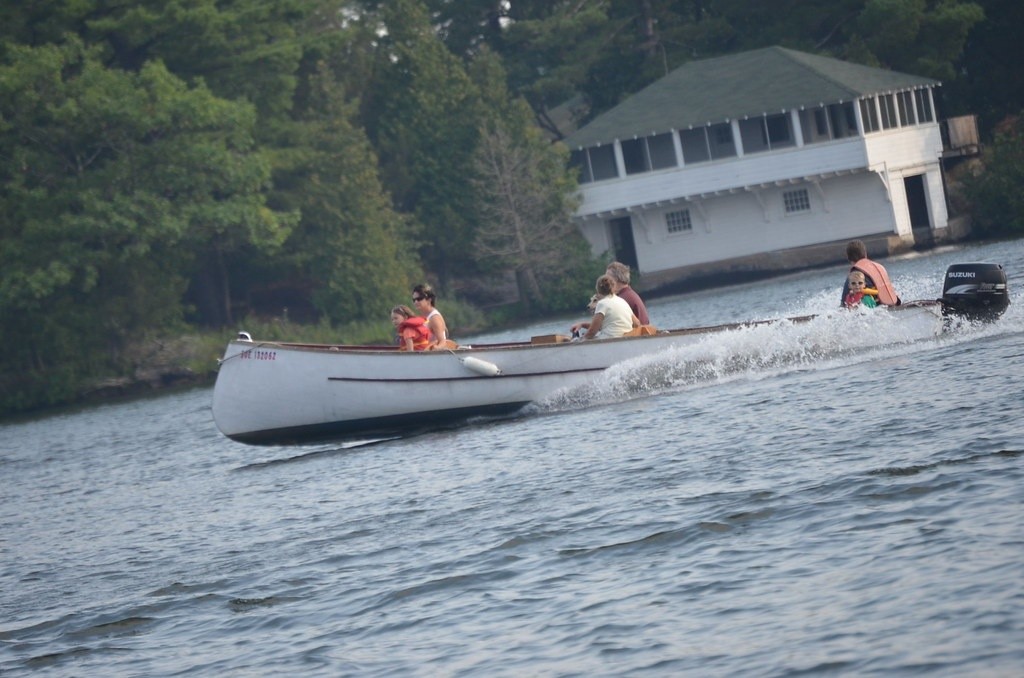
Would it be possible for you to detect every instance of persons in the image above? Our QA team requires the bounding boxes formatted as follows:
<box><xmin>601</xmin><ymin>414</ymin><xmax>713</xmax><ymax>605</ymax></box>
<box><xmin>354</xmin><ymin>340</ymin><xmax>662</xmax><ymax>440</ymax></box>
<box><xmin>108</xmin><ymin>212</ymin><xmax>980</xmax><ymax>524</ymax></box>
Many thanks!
<box><xmin>391</xmin><ymin>306</ymin><xmax>431</xmax><ymax>351</ymax></box>
<box><xmin>569</xmin><ymin>262</ymin><xmax>649</xmax><ymax>334</ymax></box>
<box><xmin>846</xmin><ymin>271</ymin><xmax>878</xmax><ymax>312</ymax></box>
<box><xmin>581</xmin><ymin>275</ymin><xmax>640</xmax><ymax>340</ymax></box>
<box><xmin>841</xmin><ymin>240</ymin><xmax>900</xmax><ymax>307</ymax></box>
<box><xmin>412</xmin><ymin>285</ymin><xmax>447</xmax><ymax>349</ymax></box>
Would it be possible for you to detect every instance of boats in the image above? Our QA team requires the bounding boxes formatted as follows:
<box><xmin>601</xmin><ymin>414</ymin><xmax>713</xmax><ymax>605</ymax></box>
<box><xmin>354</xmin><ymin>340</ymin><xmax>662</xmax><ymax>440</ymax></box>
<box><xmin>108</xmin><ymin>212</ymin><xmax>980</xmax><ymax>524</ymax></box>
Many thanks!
<box><xmin>211</xmin><ymin>263</ymin><xmax>1011</xmax><ymax>444</ymax></box>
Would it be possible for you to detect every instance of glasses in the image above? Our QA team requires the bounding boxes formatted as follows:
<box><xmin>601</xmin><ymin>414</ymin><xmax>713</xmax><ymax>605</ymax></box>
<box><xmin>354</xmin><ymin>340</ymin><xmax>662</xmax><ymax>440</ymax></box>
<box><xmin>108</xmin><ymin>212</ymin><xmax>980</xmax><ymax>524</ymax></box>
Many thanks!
<box><xmin>413</xmin><ymin>296</ymin><xmax>426</xmax><ymax>303</ymax></box>
<box><xmin>850</xmin><ymin>281</ymin><xmax>865</xmax><ymax>286</ymax></box>
